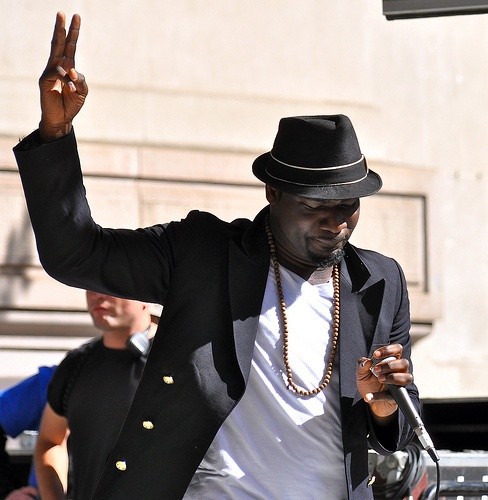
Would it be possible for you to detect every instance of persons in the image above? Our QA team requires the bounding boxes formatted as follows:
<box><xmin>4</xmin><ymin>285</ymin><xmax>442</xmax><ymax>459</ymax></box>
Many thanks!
<box><xmin>0</xmin><ymin>289</ymin><xmax>159</xmax><ymax>500</ymax></box>
<box><xmin>12</xmin><ymin>11</ymin><xmax>422</xmax><ymax>500</ymax></box>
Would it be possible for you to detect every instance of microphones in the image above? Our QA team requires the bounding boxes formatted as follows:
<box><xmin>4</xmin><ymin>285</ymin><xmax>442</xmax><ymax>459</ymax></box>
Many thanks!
<box><xmin>370</xmin><ymin>355</ymin><xmax>440</xmax><ymax>462</ymax></box>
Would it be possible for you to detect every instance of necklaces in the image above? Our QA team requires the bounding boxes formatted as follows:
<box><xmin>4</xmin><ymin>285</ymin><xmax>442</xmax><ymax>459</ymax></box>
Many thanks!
<box><xmin>268</xmin><ymin>231</ymin><xmax>340</xmax><ymax>396</ymax></box>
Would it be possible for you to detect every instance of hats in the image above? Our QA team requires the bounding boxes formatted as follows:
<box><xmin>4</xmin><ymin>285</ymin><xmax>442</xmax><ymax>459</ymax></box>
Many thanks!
<box><xmin>252</xmin><ymin>114</ymin><xmax>383</xmax><ymax>200</ymax></box>
